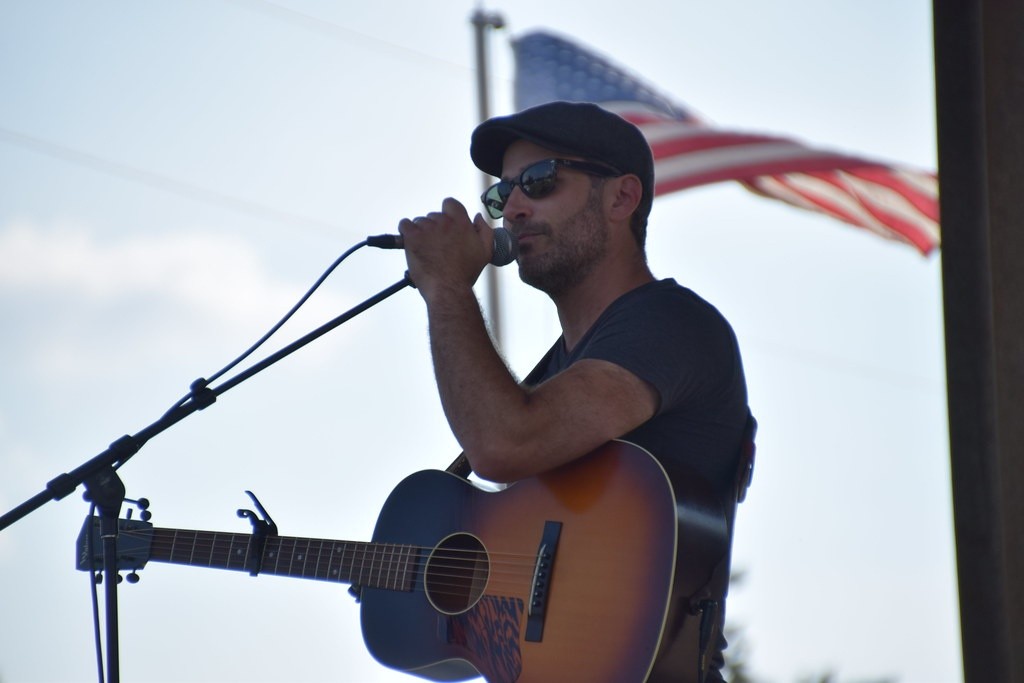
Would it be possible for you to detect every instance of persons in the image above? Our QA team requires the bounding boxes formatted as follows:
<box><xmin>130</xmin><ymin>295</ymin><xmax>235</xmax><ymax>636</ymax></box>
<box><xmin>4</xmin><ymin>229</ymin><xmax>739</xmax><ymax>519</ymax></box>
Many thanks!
<box><xmin>398</xmin><ymin>104</ymin><xmax>752</xmax><ymax>683</ymax></box>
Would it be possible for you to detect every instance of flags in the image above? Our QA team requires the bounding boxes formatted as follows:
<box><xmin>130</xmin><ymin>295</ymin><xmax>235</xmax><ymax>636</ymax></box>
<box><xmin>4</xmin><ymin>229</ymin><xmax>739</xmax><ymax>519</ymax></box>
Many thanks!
<box><xmin>510</xmin><ymin>28</ymin><xmax>940</xmax><ymax>257</ymax></box>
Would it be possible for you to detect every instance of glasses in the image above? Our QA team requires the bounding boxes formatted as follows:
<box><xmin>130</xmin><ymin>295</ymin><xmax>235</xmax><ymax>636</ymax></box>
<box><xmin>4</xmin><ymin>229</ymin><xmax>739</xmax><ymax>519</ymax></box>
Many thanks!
<box><xmin>480</xmin><ymin>158</ymin><xmax>623</xmax><ymax>219</ymax></box>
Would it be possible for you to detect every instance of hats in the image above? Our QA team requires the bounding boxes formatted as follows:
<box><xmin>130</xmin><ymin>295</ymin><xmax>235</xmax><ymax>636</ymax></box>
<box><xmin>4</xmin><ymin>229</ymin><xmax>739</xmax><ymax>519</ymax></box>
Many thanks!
<box><xmin>469</xmin><ymin>102</ymin><xmax>655</xmax><ymax>219</ymax></box>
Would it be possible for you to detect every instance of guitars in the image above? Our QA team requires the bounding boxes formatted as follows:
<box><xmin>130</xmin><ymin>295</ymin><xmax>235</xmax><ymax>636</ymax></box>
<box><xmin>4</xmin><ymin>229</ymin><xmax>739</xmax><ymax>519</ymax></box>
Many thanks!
<box><xmin>73</xmin><ymin>437</ymin><xmax>731</xmax><ymax>683</ymax></box>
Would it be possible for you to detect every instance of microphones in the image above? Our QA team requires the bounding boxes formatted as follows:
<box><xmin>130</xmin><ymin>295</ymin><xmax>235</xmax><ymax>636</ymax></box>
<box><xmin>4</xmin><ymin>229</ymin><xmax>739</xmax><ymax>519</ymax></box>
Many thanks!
<box><xmin>366</xmin><ymin>227</ymin><xmax>520</xmax><ymax>267</ymax></box>
